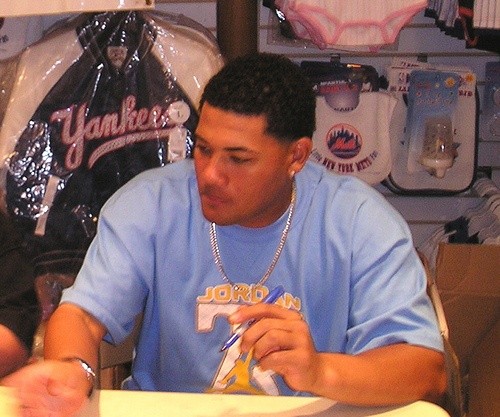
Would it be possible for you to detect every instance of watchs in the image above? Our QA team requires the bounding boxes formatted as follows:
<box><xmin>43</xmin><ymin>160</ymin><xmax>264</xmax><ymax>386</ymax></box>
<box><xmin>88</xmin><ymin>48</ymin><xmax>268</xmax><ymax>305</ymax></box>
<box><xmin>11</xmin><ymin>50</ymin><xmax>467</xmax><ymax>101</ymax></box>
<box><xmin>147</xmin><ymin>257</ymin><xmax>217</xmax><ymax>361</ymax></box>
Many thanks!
<box><xmin>57</xmin><ymin>356</ymin><xmax>97</xmax><ymax>401</ymax></box>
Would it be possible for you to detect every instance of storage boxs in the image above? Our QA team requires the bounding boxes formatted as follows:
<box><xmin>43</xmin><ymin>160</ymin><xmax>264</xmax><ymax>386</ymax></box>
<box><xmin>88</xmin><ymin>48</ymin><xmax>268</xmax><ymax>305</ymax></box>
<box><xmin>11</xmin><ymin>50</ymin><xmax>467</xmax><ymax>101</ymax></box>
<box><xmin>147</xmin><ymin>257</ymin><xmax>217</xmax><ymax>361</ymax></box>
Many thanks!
<box><xmin>413</xmin><ymin>243</ymin><xmax>500</xmax><ymax>417</ymax></box>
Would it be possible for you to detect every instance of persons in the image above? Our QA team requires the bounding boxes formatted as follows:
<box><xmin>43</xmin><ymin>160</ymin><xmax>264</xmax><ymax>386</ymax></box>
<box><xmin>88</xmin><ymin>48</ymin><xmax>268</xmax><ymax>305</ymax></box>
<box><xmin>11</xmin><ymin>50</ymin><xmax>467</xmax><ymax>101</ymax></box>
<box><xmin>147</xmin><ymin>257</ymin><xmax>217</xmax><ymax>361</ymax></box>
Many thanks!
<box><xmin>0</xmin><ymin>206</ymin><xmax>45</xmax><ymax>382</ymax></box>
<box><xmin>0</xmin><ymin>50</ymin><xmax>448</xmax><ymax>414</ymax></box>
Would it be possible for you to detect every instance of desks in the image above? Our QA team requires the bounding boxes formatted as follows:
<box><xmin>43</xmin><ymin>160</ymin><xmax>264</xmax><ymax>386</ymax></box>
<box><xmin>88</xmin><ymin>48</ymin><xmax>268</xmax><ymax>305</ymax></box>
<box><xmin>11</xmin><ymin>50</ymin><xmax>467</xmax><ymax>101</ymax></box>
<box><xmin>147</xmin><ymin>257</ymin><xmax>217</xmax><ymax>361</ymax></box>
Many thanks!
<box><xmin>0</xmin><ymin>384</ymin><xmax>446</xmax><ymax>417</ymax></box>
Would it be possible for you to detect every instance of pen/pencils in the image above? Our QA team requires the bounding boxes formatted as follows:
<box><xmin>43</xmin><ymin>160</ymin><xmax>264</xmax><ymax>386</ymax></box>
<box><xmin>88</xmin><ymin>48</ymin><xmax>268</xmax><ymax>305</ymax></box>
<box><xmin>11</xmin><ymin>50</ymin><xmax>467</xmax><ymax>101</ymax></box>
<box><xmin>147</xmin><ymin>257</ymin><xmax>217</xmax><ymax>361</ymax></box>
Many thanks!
<box><xmin>218</xmin><ymin>286</ymin><xmax>285</xmax><ymax>351</ymax></box>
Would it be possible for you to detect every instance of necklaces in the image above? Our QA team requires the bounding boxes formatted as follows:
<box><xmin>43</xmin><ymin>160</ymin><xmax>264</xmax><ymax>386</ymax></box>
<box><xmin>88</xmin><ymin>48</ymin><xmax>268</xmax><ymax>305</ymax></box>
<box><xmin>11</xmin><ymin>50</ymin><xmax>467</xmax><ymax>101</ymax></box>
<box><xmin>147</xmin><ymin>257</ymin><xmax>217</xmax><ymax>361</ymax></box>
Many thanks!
<box><xmin>207</xmin><ymin>179</ymin><xmax>298</xmax><ymax>294</ymax></box>
